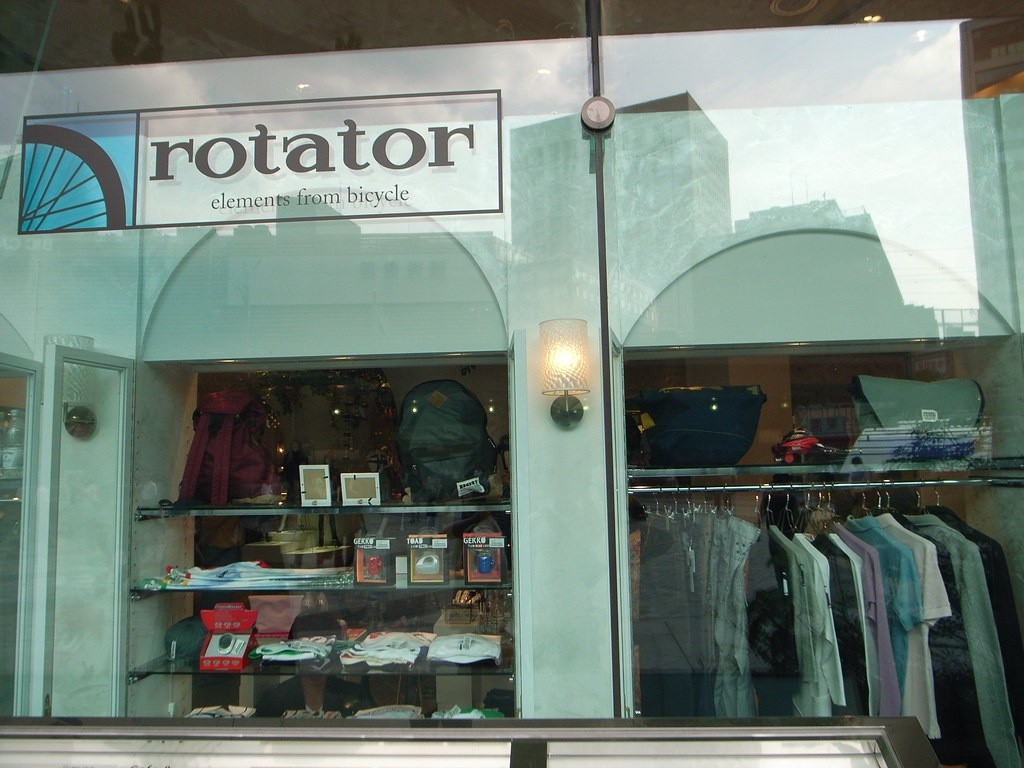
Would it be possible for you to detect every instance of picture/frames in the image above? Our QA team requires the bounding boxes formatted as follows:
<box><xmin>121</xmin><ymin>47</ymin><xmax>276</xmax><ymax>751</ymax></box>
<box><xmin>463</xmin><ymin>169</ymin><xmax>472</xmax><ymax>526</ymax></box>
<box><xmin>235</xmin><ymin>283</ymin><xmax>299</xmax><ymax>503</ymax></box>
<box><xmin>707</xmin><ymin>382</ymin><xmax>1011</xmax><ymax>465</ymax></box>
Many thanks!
<box><xmin>406</xmin><ymin>538</ymin><xmax>449</xmax><ymax>585</ymax></box>
<box><xmin>353</xmin><ymin>538</ymin><xmax>396</xmax><ymax>585</ymax></box>
<box><xmin>463</xmin><ymin>535</ymin><xmax>508</xmax><ymax>585</ymax></box>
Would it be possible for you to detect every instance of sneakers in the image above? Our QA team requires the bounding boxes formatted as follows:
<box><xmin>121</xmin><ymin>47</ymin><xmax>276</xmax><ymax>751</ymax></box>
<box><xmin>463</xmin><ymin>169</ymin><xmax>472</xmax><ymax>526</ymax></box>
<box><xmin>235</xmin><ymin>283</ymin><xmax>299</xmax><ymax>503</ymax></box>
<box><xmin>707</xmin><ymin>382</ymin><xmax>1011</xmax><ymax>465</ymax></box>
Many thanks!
<box><xmin>281</xmin><ymin>704</ymin><xmax>341</xmax><ymax>721</ymax></box>
<box><xmin>184</xmin><ymin>705</ymin><xmax>257</xmax><ymax>718</ymax></box>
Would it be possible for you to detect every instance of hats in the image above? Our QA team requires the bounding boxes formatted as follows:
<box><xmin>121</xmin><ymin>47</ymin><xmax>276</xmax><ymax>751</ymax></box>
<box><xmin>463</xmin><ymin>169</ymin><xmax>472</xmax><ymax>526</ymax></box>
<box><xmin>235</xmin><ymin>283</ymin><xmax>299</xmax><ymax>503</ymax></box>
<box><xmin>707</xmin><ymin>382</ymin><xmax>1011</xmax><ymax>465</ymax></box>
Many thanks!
<box><xmin>164</xmin><ymin>614</ymin><xmax>208</xmax><ymax>662</ymax></box>
<box><xmin>200</xmin><ymin>602</ymin><xmax>258</xmax><ymax>632</ymax></box>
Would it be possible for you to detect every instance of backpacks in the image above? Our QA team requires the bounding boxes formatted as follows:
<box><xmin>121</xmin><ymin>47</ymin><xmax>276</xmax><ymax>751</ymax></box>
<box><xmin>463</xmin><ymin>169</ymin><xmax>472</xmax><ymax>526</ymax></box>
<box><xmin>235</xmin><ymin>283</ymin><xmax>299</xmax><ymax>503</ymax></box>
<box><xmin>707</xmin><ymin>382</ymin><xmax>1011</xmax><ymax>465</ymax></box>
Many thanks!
<box><xmin>393</xmin><ymin>377</ymin><xmax>509</xmax><ymax>502</ymax></box>
<box><xmin>172</xmin><ymin>385</ymin><xmax>284</xmax><ymax>506</ymax></box>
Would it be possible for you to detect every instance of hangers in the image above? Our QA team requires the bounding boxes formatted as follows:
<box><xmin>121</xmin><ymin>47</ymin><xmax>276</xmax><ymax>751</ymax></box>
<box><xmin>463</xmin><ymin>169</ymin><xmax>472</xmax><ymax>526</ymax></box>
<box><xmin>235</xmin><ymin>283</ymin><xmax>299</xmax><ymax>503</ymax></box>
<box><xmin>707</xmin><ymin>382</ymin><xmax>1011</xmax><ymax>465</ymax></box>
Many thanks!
<box><xmin>637</xmin><ymin>477</ymin><xmax>1001</xmax><ymax>566</ymax></box>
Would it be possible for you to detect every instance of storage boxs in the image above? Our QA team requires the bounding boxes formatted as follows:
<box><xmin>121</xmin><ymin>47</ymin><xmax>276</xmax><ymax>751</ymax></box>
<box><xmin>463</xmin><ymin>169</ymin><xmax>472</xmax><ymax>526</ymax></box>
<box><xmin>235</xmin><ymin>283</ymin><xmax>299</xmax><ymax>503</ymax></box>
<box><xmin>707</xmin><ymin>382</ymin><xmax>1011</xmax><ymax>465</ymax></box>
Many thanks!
<box><xmin>199</xmin><ymin>603</ymin><xmax>260</xmax><ymax>670</ymax></box>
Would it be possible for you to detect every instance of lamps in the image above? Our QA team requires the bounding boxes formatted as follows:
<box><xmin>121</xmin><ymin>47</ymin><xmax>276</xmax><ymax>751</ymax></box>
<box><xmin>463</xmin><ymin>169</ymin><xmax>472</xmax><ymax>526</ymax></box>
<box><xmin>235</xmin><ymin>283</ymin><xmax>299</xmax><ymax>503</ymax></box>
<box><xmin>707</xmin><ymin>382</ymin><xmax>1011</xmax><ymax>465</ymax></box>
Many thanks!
<box><xmin>540</xmin><ymin>318</ymin><xmax>591</xmax><ymax>428</ymax></box>
<box><xmin>39</xmin><ymin>334</ymin><xmax>97</xmax><ymax>436</ymax></box>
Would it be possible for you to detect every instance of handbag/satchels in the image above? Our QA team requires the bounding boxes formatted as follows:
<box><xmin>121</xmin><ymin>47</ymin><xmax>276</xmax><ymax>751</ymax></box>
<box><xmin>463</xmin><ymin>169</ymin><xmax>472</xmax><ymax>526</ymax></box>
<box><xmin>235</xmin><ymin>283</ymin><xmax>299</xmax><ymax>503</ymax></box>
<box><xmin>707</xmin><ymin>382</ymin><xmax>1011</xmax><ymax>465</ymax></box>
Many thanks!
<box><xmin>339</xmin><ymin>632</ymin><xmax>421</xmax><ymax>667</ymax></box>
<box><xmin>851</xmin><ymin>374</ymin><xmax>984</xmax><ymax>435</ymax></box>
<box><xmin>284</xmin><ymin>440</ymin><xmax>309</xmax><ymax>479</ymax></box>
<box><xmin>247</xmin><ymin>640</ymin><xmax>333</xmax><ymax>661</ymax></box>
<box><xmin>425</xmin><ymin>632</ymin><xmax>503</xmax><ymax>665</ymax></box>
<box><xmin>635</xmin><ymin>382</ymin><xmax>767</xmax><ymax>468</ymax></box>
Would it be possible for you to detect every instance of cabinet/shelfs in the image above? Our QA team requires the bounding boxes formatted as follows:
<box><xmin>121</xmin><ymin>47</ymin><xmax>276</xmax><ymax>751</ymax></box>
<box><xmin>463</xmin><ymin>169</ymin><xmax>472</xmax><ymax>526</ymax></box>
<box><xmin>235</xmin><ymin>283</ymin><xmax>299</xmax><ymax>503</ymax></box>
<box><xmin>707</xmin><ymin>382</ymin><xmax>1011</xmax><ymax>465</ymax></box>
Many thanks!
<box><xmin>127</xmin><ymin>503</ymin><xmax>516</xmax><ymax>720</ymax></box>
